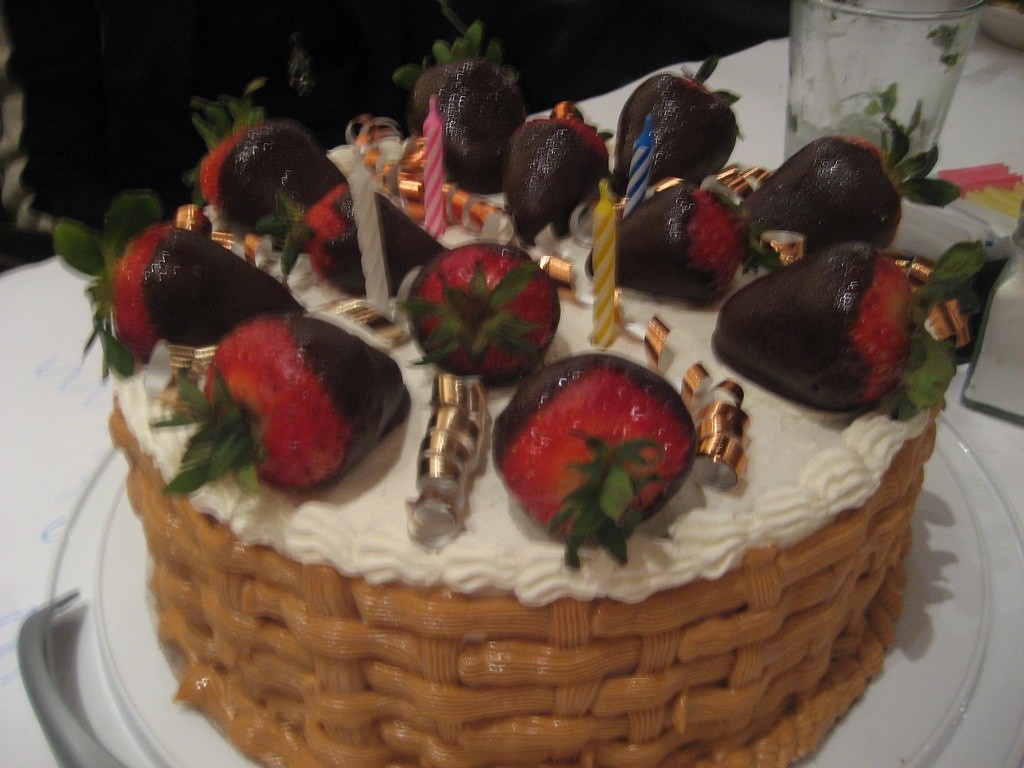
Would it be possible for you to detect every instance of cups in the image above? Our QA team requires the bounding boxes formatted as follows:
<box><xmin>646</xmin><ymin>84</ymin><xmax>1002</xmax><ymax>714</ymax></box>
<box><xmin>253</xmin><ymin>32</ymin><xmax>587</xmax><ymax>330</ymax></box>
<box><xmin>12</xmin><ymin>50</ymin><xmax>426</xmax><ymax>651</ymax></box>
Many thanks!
<box><xmin>784</xmin><ymin>0</ymin><xmax>983</xmax><ymax>196</ymax></box>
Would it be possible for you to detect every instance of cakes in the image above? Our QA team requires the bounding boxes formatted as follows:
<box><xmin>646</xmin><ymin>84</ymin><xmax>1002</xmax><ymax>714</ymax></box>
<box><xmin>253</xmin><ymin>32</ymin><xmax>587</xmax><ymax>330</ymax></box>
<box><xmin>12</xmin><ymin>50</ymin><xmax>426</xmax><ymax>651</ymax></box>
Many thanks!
<box><xmin>51</xmin><ymin>21</ymin><xmax>988</xmax><ymax>768</ymax></box>
